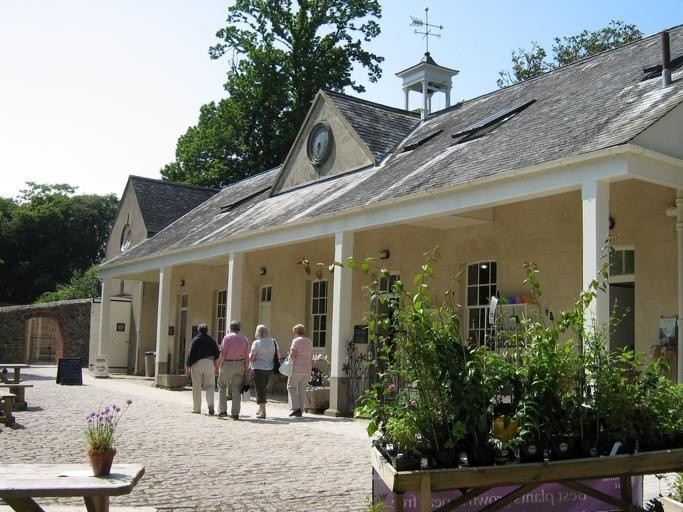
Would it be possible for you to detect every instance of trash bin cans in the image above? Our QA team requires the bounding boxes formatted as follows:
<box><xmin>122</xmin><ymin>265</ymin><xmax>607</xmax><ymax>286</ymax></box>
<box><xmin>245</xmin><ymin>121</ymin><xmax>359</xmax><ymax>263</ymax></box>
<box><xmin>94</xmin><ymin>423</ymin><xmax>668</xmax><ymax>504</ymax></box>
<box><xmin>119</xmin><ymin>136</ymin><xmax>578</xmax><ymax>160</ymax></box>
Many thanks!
<box><xmin>144</xmin><ymin>352</ymin><xmax>154</xmax><ymax>377</ymax></box>
<box><xmin>93</xmin><ymin>355</ymin><xmax>108</xmax><ymax>377</ymax></box>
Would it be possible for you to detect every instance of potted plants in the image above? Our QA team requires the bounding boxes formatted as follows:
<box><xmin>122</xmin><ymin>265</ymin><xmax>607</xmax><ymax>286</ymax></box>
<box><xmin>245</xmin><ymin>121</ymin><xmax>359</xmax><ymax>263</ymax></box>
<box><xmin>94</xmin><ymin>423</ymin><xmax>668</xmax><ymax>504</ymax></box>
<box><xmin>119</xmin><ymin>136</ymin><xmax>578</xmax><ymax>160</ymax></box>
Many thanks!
<box><xmin>326</xmin><ymin>259</ymin><xmax>683</xmax><ymax>468</ymax></box>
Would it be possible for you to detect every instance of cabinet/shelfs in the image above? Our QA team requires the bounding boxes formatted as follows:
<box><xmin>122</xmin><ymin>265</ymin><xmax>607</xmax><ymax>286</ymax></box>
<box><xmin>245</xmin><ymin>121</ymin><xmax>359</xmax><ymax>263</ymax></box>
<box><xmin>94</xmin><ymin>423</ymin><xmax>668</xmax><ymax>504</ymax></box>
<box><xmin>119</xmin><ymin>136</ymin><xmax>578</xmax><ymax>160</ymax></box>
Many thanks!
<box><xmin>371</xmin><ymin>439</ymin><xmax>681</xmax><ymax>508</ymax></box>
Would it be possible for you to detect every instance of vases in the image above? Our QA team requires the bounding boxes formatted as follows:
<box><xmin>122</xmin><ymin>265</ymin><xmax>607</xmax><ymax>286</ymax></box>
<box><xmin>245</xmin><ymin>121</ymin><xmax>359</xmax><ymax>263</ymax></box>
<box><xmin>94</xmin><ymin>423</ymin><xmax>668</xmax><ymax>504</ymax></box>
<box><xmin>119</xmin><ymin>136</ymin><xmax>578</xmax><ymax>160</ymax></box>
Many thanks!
<box><xmin>88</xmin><ymin>449</ymin><xmax>117</xmax><ymax>476</ymax></box>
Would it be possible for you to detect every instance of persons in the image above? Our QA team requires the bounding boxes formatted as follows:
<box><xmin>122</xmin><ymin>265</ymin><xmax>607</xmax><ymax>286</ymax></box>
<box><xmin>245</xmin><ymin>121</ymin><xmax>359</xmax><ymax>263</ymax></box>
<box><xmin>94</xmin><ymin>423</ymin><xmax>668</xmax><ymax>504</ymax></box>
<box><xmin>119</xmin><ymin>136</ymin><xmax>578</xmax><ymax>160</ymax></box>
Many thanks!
<box><xmin>187</xmin><ymin>320</ymin><xmax>313</xmax><ymax>420</ymax></box>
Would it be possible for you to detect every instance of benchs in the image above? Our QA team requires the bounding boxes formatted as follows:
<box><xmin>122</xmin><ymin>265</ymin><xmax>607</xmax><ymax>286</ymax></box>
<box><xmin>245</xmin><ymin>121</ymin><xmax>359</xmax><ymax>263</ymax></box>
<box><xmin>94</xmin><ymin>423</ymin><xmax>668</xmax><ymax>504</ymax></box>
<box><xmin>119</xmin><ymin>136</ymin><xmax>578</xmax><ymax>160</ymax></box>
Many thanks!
<box><xmin>0</xmin><ymin>364</ymin><xmax>33</xmax><ymax>427</ymax></box>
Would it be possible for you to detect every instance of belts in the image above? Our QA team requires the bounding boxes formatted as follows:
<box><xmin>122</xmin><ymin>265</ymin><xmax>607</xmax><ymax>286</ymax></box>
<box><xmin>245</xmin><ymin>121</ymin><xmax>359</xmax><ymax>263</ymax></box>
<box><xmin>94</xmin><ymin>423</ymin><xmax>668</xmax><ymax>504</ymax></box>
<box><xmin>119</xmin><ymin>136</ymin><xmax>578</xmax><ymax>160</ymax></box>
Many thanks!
<box><xmin>226</xmin><ymin>359</ymin><xmax>244</xmax><ymax>361</ymax></box>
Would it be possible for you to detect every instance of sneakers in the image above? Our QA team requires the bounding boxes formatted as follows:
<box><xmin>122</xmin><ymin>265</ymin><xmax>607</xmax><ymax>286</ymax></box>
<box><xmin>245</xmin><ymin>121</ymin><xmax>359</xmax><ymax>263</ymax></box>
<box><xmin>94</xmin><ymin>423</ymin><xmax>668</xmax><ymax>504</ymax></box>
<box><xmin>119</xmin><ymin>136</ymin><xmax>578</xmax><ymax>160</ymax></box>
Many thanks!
<box><xmin>191</xmin><ymin>406</ymin><xmax>238</xmax><ymax>420</ymax></box>
<box><xmin>289</xmin><ymin>408</ymin><xmax>302</xmax><ymax>417</ymax></box>
<box><xmin>256</xmin><ymin>409</ymin><xmax>266</xmax><ymax>418</ymax></box>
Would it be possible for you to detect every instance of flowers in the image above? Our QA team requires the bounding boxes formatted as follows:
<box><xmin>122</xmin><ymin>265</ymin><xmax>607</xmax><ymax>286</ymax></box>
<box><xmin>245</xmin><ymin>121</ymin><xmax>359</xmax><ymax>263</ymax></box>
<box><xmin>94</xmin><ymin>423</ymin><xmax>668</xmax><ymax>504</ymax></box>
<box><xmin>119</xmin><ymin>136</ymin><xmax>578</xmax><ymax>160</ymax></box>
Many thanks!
<box><xmin>84</xmin><ymin>399</ymin><xmax>133</xmax><ymax>452</ymax></box>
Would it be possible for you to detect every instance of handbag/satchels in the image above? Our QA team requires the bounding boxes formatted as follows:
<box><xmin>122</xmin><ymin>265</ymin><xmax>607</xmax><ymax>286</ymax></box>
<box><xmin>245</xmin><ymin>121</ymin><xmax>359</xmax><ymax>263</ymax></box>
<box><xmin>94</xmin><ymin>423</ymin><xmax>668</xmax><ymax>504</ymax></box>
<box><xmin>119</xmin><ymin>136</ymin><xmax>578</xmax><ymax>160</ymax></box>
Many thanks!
<box><xmin>279</xmin><ymin>355</ymin><xmax>293</xmax><ymax>377</ymax></box>
<box><xmin>273</xmin><ymin>340</ymin><xmax>281</xmax><ymax>374</ymax></box>
